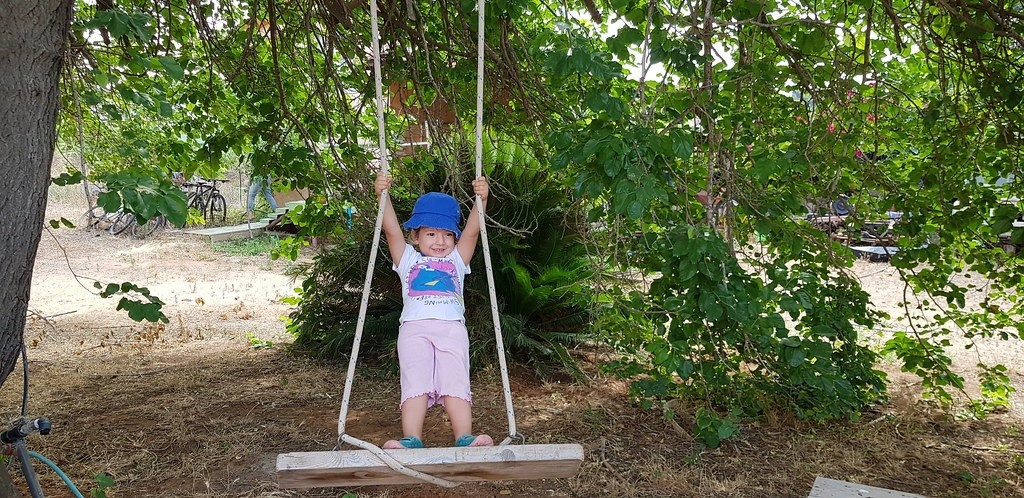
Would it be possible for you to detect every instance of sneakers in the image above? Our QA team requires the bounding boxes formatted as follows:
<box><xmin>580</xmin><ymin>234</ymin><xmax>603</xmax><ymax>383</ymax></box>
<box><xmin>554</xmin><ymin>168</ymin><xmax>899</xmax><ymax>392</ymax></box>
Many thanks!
<box><xmin>382</xmin><ymin>435</ymin><xmax>423</xmax><ymax>448</ymax></box>
<box><xmin>454</xmin><ymin>433</ymin><xmax>494</xmax><ymax>447</ymax></box>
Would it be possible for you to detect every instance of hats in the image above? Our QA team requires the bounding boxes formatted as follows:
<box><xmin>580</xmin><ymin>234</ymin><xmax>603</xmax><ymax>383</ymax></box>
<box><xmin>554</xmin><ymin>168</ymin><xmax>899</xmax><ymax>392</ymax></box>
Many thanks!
<box><xmin>403</xmin><ymin>192</ymin><xmax>462</xmax><ymax>239</ymax></box>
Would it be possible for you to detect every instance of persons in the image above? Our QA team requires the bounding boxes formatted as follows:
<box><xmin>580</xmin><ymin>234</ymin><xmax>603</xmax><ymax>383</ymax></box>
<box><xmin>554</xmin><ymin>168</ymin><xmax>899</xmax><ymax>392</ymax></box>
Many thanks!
<box><xmin>375</xmin><ymin>171</ymin><xmax>494</xmax><ymax>449</ymax></box>
<box><xmin>244</xmin><ymin>172</ymin><xmax>278</xmax><ymax>222</ymax></box>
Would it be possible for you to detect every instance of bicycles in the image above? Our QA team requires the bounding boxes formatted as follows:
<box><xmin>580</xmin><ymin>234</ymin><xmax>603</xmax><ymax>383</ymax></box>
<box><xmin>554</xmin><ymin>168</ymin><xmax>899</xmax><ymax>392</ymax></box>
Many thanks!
<box><xmin>79</xmin><ymin>174</ymin><xmax>230</xmax><ymax>239</ymax></box>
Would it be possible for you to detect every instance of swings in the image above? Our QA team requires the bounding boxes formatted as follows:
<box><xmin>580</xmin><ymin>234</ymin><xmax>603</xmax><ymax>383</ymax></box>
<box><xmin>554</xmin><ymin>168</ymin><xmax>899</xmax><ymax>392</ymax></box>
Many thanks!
<box><xmin>278</xmin><ymin>0</ymin><xmax>584</xmax><ymax>486</ymax></box>
